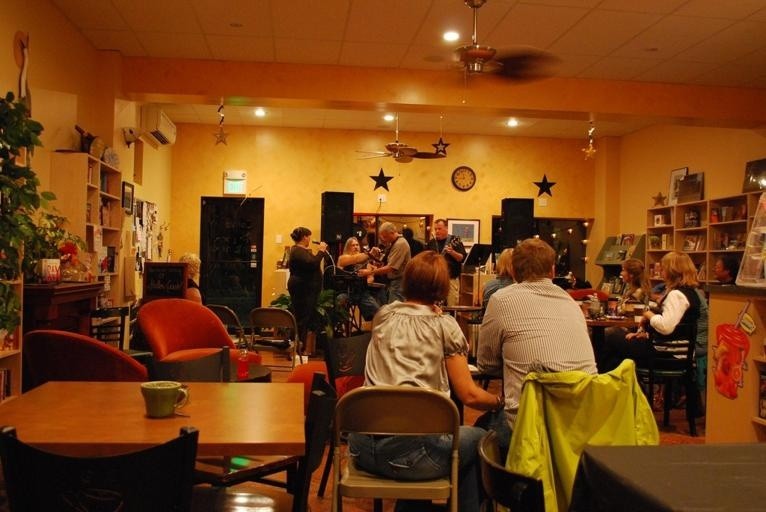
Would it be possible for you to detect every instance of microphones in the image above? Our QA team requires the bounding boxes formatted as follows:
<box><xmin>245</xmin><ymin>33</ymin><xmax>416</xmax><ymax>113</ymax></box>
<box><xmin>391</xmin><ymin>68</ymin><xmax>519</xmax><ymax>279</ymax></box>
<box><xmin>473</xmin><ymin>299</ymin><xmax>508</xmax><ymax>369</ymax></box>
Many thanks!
<box><xmin>311</xmin><ymin>240</ymin><xmax>335</xmax><ymax>273</ymax></box>
<box><xmin>425</xmin><ymin>225</ymin><xmax>441</xmax><ymax>257</ymax></box>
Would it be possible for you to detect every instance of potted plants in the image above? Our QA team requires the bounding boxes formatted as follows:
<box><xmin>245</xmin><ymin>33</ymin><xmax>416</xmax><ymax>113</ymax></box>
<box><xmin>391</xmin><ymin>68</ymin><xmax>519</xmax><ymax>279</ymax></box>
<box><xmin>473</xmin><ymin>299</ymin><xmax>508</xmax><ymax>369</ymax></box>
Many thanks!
<box><xmin>272</xmin><ymin>289</ymin><xmax>350</xmax><ymax>357</ymax></box>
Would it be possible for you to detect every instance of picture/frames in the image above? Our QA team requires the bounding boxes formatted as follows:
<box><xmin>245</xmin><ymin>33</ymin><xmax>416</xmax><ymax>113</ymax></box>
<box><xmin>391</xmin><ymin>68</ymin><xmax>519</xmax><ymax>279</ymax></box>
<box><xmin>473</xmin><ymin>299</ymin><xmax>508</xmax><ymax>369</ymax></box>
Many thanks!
<box><xmin>122</xmin><ymin>181</ymin><xmax>134</xmax><ymax>215</ymax></box>
<box><xmin>667</xmin><ymin>167</ymin><xmax>690</xmax><ymax>206</ymax></box>
<box><xmin>447</xmin><ymin>219</ymin><xmax>480</xmax><ymax>247</ymax></box>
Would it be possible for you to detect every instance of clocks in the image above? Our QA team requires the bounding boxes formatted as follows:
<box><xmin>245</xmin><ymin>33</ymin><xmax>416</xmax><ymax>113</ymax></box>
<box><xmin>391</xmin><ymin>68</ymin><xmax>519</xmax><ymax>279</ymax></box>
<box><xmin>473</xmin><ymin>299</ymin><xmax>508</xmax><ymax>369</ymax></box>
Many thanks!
<box><xmin>451</xmin><ymin>166</ymin><xmax>478</xmax><ymax>191</ymax></box>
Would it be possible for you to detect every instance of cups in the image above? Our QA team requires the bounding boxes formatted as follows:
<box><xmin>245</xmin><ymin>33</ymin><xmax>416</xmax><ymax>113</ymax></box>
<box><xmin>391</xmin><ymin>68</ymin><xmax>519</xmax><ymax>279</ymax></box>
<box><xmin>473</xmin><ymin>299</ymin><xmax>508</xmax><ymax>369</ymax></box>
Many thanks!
<box><xmin>141</xmin><ymin>382</ymin><xmax>188</xmax><ymax>419</ymax></box>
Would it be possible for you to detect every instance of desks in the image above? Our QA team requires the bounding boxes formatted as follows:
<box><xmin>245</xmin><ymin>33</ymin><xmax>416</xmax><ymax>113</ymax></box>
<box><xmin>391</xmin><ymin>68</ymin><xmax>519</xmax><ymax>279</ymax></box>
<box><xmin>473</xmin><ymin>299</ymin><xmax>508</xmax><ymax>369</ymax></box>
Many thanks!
<box><xmin>463</xmin><ymin>357</ymin><xmax>504</xmax><ymax>391</ymax></box>
<box><xmin>586</xmin><ymin>315</ymin><xmax>634</xmax><ymax>375</ymax></box>
<box><xmin>569</xmin><ymin>442</ymin><xmax>766</xmax><ymax>511</ymax></box>
<box><xmin>0</xmin><ymin>381</ymin><xmax>307</xmax><ymax>487</ymax></box>
<box><xmin>440</xmin><ymin>304</ymin><xmax>484</xmax><ymax>321</ymax></box>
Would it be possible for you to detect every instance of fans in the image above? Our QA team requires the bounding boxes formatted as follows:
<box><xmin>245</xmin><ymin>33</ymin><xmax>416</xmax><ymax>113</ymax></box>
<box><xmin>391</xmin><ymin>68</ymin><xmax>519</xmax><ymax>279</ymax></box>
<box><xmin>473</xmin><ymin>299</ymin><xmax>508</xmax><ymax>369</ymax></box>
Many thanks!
<box><xmin>354</xmin><ymin>112</ymin><xmax>445</xmax><ymax>163</ymax></box>
<box><xmin>390</xmin><ymin>0</ymin><xmax>562</xmax><ymax>84</ymax></box>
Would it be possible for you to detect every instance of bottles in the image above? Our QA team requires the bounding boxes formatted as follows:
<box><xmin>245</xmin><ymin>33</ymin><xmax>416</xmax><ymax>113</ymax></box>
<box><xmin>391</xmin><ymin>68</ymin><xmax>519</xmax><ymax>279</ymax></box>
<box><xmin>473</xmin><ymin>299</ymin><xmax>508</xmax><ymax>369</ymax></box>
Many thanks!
<box><xmin>591</xmin><ymin>292</ymin><xmax>599</xmax><ymax>320</ymax></box>
<box><xmin>237</xmin><ymin>344</ymin><xmax>250</xmax><ymax>379</ymax></box>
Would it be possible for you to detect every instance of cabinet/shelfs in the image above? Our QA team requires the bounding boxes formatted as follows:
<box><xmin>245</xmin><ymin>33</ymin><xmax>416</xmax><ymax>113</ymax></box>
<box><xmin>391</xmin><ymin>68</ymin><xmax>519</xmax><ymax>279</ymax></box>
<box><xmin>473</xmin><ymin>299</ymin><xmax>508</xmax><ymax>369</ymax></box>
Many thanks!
<box><xmin>643</xmin><ymin>191</ymin><xmax>766</xmax><ymax>295</ymax></box>
<box><xmin>704</xmin><ymin>283</ymin><xmax>766</xmax><ymax>443</ymax></box>
<box><xmin>198</xmin><ymin>195</ymin><xmax>265</xmax><ymax>336</ymax></box>
<box><xmin>49</xmin><ymin>151</ymin><xmax>123</xmax><ymax>307</ymax></box>
<box><xmin>0</xmin><ymin>239</ymin><xmax>22</xmax><ymax>404</ymax></box>
<box><xmin>458</xmin><ymin>273</ymin><xmax>496</xmax><ymax>357</ymax></box>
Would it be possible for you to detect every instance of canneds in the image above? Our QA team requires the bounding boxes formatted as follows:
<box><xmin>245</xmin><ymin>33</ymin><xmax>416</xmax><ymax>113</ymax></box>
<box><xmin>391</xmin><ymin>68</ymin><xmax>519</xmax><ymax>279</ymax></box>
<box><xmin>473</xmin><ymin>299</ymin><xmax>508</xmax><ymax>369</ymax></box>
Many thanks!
<box><xmin>607</xmin><ymin>297</ymin><xmax>619</xmax><ymax>316</ymax></box>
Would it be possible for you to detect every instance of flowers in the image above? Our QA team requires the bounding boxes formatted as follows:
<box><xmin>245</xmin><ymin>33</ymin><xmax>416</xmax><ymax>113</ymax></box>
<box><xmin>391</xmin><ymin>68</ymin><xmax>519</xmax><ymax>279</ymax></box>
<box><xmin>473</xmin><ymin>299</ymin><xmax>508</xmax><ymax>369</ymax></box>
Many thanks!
<box><xmin>29</xmin><ymin>212</ymin><xmax>85</xmax><ymax>259</ymax></box>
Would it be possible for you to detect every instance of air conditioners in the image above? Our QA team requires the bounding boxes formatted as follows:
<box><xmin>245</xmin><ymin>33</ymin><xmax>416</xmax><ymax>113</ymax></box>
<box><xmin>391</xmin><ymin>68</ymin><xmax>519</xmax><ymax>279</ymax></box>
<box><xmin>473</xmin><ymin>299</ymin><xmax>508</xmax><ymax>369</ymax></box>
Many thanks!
<box><xmin>140</xmin><ymin>105</ymin><xmax>178</xmax><ymax>150</ymax></box>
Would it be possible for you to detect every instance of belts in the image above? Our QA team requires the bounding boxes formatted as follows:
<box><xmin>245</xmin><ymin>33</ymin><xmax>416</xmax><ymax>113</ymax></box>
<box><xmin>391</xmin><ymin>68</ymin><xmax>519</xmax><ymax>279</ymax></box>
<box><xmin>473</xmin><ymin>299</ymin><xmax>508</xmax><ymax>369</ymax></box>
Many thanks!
<box><xmin>366</xmin><ymin>434</ymin><xmax>394</xmax><ymax>442</ymax></box>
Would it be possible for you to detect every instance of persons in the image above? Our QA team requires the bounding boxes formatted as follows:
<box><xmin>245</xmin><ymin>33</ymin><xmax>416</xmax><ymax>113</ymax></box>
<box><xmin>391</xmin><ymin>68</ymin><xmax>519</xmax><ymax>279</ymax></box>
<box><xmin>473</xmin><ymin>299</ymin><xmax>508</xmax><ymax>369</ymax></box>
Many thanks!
<box><xmin>712</xmin><ymin>254</ymin><xmax>740</xmax><ymax>288</ymax></box>
<box><xmin>619</xmin><ymin>257</ymin><xmax>653</xmax><ymax>304</ymax></box>
<box><xmin>178</xmin><ymin>252</ymin><xmax>202</xmax><ymax>303</ymax></box>
<box><xmin>337</xmin><ymin>235</ymin><xmax>386</xmax><ymax>277</ymax></box>
<box><xmin>605</xmin><ymin>250</ymin><xmax>698</xmax><ymax>375</ymax></box>
<box><xmin>286</xmin><ymin>225</ymin><xmax>329</xmax><ymax>356</ymax></box>
<box><xmin>424</xmin><ymin>217</ymin><xmax>467</xmax><ymax>316</ymax></box>
<box><xmin>345</xmin><ymin>249</ymin><xmax>504</xmax><ymax>511</ymax></box>
<box><xmin>476</xmin><ymin>238</ymin><xmax>601</xmax><ymax>459</ymax></box>
<box><xmin>356</xmin><ymin>220</ymin><xmax>415</xmax><ymax>303</ymax></box>
<box><xmin>649</xmin><ymin>281</ymin><xmax>707</xmax><ymax>407</ymax></box>
<box><xmin>481</xmin><ymin>246</ymin><xmax>518</xmax><ymax>313</ymax></box>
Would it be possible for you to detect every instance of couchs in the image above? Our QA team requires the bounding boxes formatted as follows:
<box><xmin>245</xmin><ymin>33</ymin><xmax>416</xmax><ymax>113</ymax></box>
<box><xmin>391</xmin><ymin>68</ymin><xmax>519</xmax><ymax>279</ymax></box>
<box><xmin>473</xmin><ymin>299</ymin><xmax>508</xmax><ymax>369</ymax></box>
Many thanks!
<box><xmin>24</xmin><ymin>330</ymin><xmax>148</xmax><ymax>382</ymax></box>
<box><xmin>139</xmin><ymin>296</ymin><xmax>273</xmax><ymax>382</ymax></box>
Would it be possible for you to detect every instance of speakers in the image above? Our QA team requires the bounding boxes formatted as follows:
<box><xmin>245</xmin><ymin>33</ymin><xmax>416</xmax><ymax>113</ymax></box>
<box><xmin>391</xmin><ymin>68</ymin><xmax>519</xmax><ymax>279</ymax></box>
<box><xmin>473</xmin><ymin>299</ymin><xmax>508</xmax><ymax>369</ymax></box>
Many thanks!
<box><xmin>498</xmin><ymin>197</ymin><xmax>537</xmax><ymax>237</ymax></box>
<box><xmin>319</xmin><ymin>190</ymin><xmax>354</xmax><ymax>243</ymax></box>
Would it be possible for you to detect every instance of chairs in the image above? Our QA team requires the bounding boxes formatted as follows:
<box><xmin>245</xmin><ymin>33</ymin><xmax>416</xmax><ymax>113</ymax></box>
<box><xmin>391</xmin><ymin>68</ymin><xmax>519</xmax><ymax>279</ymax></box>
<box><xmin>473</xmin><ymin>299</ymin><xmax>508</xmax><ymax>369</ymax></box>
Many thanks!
<box><xmin>87</xmin><ymin>306</ymin><xmax>130</xmax><ymax>351</ymax></box>
<box><xmin>316</xmin><ymin>332</ymin><xmax>374</xmax><ymax>498</ymax></box>
<box><xmin>635</xmin><ymin>324</ymin><xmax>698</xmax><ymax>437</ymax></box>
<box><xmin>0</xmin><ymin>424</ymin><xmax>201</xmax><ymax>512</ymax></box>
<box><xmin>204</xmin><ymin>304</ymin><xmax>250</xmax><ymax>351</ymax></box>
<box><xmin>478</xmin><ymin>430</ymin><xmax>547</xmax><ymax>512</ymax></box>
<box><xmin>196</xmin><ymin>371</ymin><xmax>331</xmax><ymax>512</ymax></box>
<box><xmin>332</xmin><ymin>386</ymin><xmax>460</xmax><ymax>512</ymax></box>
<box><xmin>246</xmin><ymin>307</ymin><xmax>303</xmax><ymax>372</ymax></box>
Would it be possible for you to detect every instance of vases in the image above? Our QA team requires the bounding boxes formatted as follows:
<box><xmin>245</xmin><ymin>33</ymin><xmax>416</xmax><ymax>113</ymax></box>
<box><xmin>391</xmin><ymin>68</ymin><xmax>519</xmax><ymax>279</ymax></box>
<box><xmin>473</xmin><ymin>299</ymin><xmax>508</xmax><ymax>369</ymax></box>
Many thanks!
<box><xmin>39</xmin><ymin>259</ymin><xmax>61</xmax><ymax>283</ymax></box>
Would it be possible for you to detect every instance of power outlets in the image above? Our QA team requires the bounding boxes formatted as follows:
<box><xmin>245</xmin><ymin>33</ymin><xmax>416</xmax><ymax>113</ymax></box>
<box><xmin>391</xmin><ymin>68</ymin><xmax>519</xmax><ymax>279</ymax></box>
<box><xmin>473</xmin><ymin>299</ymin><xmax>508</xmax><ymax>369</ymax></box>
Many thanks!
<box><xmin>376</xmin><ymin>193</ymin><xmax>387</xmax><ymax>203</ymax></box>
